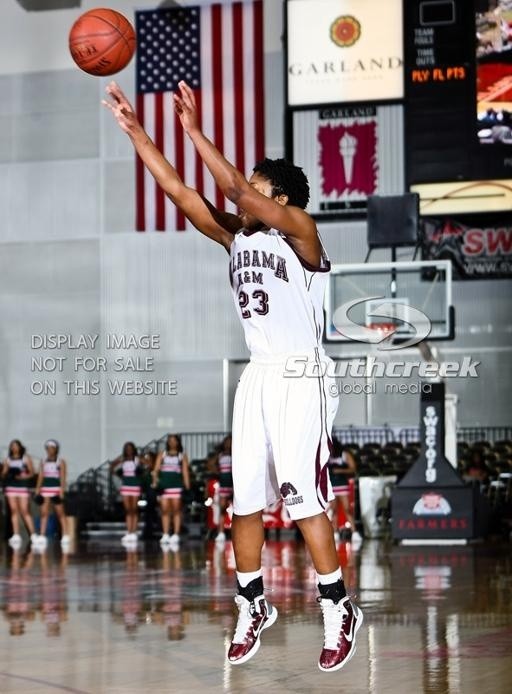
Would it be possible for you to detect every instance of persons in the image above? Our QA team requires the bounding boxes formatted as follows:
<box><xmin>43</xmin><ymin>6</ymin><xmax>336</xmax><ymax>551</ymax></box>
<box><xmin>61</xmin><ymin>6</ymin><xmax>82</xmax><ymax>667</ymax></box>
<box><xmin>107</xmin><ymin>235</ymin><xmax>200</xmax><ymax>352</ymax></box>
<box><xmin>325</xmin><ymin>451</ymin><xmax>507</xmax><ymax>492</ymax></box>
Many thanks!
<box><xmin>1</xmin><ymin>439</ymin><xmax>40</xmax><ymax>545</ymax></box>
<box><xmin>112</xmin><ymin>543</ymin><xmax>148</xmax><ymax>637</ymax></box>
<box><xmin>95</xmin><ymin>77</ymin><xmax>368</xmax><ymax>675</ymax></box>
<box><xmin>153</xmin><ymin>545</ymin><xmax>190</xmax><ymax>642</ymax></box>
<box><xmin>28</xmin><ymin>439</ymin><xmax>73</xmax><ymax>544</ymax></box>
<box><xmin>111</xmin><ymin>432</ymin><xmax>366</xmax><ymax>544</ymax></box>
<box><xmin>1</xmin><ymin>545</ymin><xmax>73</xmax><ymax>639</ymax></box>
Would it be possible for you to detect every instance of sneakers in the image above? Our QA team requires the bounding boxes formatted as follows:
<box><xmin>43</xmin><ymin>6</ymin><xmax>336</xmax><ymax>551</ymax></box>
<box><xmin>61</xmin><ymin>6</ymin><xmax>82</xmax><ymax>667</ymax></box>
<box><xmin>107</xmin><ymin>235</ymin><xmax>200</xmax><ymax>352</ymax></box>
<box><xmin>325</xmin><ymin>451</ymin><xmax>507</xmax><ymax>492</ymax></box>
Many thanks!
<box><xmin>8</xmin><ymin>533</ymin><xmax>72</xmax><ymax>545</ymax></box>
<box><xmin>318</xmin><ymin>595</ymin><xmax>364</xmax><ymax>672</ymax></box>
<box><xmin>214</xmin><ymin>532</ymin><xmax>226</xmax><ymax>542</ymax></box>
<box><xmin>227</xmin><ymin>592</ymin><xmax>279</xmax><ymax>666</ymax></box>
<box><xmin>334</xmin><ymin>531</ymin><xmax>363</xmax><ymax>541</ymax></box>
<box><xmin>119</xmin><ymin>531</ymin><xmax>180</xmax><ymax>543</ymax></box>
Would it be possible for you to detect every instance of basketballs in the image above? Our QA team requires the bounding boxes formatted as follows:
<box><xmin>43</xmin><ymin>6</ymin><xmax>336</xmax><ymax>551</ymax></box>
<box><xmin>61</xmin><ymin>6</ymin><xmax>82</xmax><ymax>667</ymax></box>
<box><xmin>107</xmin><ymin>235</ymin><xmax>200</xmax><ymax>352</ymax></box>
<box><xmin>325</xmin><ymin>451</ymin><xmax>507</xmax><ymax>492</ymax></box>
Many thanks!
<box><xmin>69</xmin><ymin>8</ymin><xmax>136</xmax><ymax>76</ymax></box>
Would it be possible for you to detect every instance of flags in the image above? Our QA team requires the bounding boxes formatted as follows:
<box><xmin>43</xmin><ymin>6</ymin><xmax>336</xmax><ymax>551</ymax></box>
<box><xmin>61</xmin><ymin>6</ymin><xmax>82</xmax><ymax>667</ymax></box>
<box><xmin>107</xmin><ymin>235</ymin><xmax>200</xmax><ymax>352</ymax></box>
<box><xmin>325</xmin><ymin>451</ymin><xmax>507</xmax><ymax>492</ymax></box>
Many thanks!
<box><xmin>132</xmin><ymin>0</ymin><xmax>270</xmax><ymax>235</ymax></box>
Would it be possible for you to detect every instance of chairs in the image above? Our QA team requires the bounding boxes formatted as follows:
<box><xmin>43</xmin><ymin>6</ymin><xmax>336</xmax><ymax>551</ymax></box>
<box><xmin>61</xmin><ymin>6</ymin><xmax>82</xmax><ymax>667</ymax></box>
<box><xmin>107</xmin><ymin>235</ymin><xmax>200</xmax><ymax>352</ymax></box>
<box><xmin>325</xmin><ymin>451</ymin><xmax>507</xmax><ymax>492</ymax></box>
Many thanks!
<box><xmin>346</xmin><ymin>439</ymin><xmax>511</xmax><ymax>482</ymax></box>
<box><xmin>183</xmin><ymin>458</ymin><xmax>211</xmax><ymax>537</ymax></box>
